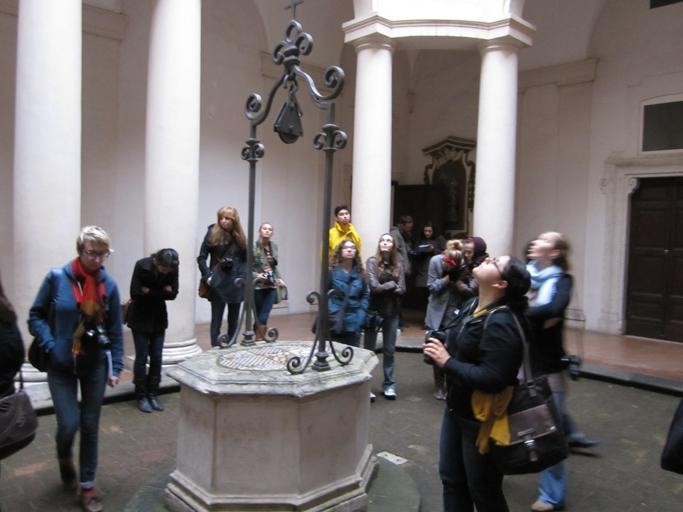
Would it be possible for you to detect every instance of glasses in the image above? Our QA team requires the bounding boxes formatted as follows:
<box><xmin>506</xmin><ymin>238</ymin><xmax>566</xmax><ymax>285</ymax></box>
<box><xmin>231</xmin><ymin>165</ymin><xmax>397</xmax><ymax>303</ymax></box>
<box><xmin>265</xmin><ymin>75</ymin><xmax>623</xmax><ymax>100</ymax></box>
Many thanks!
<box><xmin>83</xmin><ymin>249</ymin><xmax>111</xmax><ymax>259</ymax></box>
<box><xmin>491</xmin><ymin>256</ymin><xmax>505</xmax><ymax>281</ymax></box>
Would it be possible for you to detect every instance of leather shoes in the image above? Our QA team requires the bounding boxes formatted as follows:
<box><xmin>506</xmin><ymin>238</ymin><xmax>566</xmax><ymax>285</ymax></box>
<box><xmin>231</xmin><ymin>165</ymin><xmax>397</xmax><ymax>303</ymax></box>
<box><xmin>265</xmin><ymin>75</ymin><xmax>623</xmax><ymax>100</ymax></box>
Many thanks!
<box><xmin>136</xmin><ymin>396</ymin><xmax>153</xmax><ymax>413</ymax></box>
<box><xmin>368</xmin><ymin>390</ymin><xmax>376</xmax><ymax>399</ymax></box>
<box><xmin>570</xmin><ymin>432</ymin><xmax>602</xmax><ymax>452</ymax></box>
<box><xmin>384</xmin><ymin>385</ymin><xmax>398</xmax><ymax>399</ymax></box>
<box><xmin>531</xmin><ymin>498</ymin><xmax>553</xmax><ymax>511</ymax></box>
<box><xmin>150</xmin><ymin>394</ymin><xmax>164</xmax><ymax>412</ymax></box>
<box><xmin>82</xmin><ymin>492</ymin><xmax>103</xmax><ymax>512</ymax></box>
<box><xmin>62</xmin><ymin>467</ymin><xmax>79</xmax><ymax>492</ymax></box>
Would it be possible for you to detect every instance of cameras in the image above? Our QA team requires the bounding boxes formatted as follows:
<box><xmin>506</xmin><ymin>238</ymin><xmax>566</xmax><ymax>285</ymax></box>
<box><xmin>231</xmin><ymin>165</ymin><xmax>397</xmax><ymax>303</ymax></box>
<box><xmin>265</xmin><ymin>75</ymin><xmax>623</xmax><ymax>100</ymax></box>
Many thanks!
<box><xmin>424</xmin><ymin>330</ymin><xmax>448</xmax><ymax>366</ymax></box>
<box><xmin>560</xmin><ymin>354</ymin><xmax>582</xmax><ymax>381</ymax></box>
<box><xmin>220</xmin><ymin>257</ymin><xmax>234</xmax><ymax>273</ymax></box>
<box><xmin>81</xmin><ymin>321</ymin><xmax>112</xmax><ymax>352</ymax></box>
<box><xmin>263</xmin><ymin>270</ymin><xmax>275</xmax><ymax>285</ymax></box>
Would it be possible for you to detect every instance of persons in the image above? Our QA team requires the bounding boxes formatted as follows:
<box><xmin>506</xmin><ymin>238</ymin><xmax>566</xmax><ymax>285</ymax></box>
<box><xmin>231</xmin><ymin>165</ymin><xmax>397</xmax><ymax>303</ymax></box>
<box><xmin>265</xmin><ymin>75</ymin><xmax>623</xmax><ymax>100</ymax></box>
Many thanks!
<box><xmin>0</xmin><ymin>281</ymin><xmax>26</xmax><ymax>400</ymax></box>
<box><xmin>422</xmin><ymin>255</ymin><xmax>532</xmax><ymax>512</ymax></box>
<box><xmin>463</xmin><ymin>236</ymin><xmax>489</xmax><ymax>298</ymax></box>
<box><xmin>366</xmin><ymin>233</ymin><xmax>406</xmax><ymax>400</ymax></box>
<box><xmin>127</xmin><ymin>248</ymin><xmax>180</xmax><ymax>412</ymax></box>
<box><xmin>524</xmin><ymin>238</ymin><xmax>602</xmax><ymax>449</ymax></box>
<box><xmin>196</xmin><ymin>206</ymin><xmax>259</xmax><ymax>347</ymax></box>
<box><xmin>519</xmin><ymin>231</ymin><xmax>573</xmax><ymax>512</ymax></box>
<box><xmin>319</xmin><ymin>205</ymin><xmax>361</xmax><ymax>269</ymax></box>
<box><xmin>251</xmin><ymin>222</ymin><xmax>278</xmax><ymax>341</ymax></box>
<box><xmin>424</xmin><ymin>238</ymin><xmax>463</xmax><ymax>401</ymax></box>
<box><xmin>26</xmin><ymin>225</ymin><xmax>125</xmax><ymax>512</ymax></box>
<box><xmin>415</xmin><ymin>219</ymin><xmax>447</xmax><ymax>319</ymax></box>
<box><xmin>390</xmin><ymin>215</ymin><xmax>413</xmax><ymax>326</ymax></box>
<box><xmin>328</xmin><ymin>239</ymin><xmax>370</xmax><ymax>348</ymax></box>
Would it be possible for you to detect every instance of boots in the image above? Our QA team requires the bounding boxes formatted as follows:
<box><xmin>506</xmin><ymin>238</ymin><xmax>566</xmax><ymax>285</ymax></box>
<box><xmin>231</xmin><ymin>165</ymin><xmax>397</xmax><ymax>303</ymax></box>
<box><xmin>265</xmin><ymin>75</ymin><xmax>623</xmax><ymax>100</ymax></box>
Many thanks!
<box><xmin>433</xmin><ymin>374</ymin><xmax>444</xmax><ymax>400</ymax></box>
<box><xmin>256</xmin><ymin>324</ymin><xmax>269</xmax><ymax>341</ymax></box>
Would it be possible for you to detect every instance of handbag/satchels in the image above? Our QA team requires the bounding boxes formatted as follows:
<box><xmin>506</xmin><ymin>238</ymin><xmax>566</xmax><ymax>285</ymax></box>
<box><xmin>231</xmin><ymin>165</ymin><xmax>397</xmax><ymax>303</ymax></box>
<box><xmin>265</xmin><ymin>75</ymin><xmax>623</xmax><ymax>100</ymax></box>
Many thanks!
<box><xmin>0</xmin><ymin>366</ymin><xmax>40</xmax><ymax>458</ymax></box>
<box><xmin>274</xmin><ymin>271</ymin><xmax>289</xmax><ymax>302</ymax></box>
<box><xmin>122</xmin><ymin>298</ymin><xmax>136</xmax><ymax>325</ymax></box>
<box><xmin>199</xmin><ymin>242</ymin><xmax>235</xmax><ymax>299</ymax></box>
<box><xmin>311</xmin><ymin>283</ymin><xmax>352</xmax><ymax>335</ymax></box>
<box><xmin>478</xmin><ymin>306</ymin><xmax>569</xmax><ymax>475</ymax></box>
